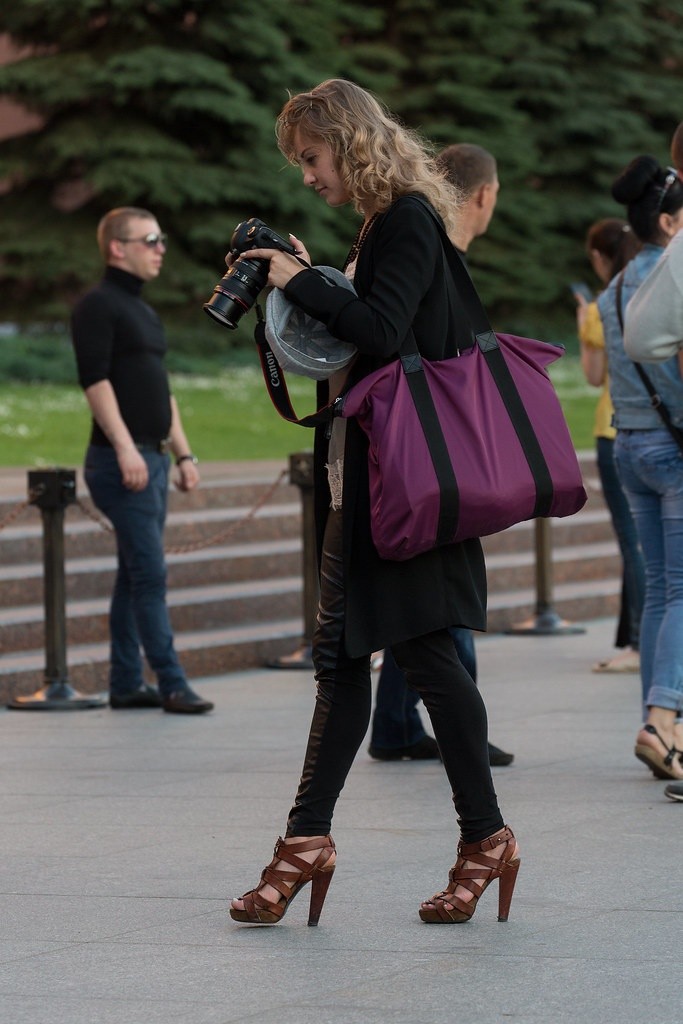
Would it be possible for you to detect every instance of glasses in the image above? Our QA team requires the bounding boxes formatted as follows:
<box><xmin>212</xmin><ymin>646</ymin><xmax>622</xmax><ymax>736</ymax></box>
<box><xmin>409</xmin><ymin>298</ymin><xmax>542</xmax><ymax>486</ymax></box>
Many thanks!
<box><xmin>653</xmin><ymin>166</ymin><xmax>679</xmax><ymax>212</ymax></box>
<box><xmin>115</xmin><ymin>233</ymin><xmax>167</xmax><ymax>247</ymax></box>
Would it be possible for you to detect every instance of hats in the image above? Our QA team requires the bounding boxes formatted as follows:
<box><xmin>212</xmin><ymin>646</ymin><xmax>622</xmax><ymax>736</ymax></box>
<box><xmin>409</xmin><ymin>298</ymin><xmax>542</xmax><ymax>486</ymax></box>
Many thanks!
<box><xmin>261</xmin><ymin>265</ymin><xmax>360</xmax><ymax>379</ymax></box>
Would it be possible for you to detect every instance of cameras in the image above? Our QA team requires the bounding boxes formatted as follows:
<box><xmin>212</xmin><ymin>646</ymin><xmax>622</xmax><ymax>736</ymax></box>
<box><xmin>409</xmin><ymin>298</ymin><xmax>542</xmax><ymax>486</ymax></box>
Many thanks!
<box><xmin>204</xmin><ymin>218</ymin><xmax>298</xmax><ymax>329</ymax></box>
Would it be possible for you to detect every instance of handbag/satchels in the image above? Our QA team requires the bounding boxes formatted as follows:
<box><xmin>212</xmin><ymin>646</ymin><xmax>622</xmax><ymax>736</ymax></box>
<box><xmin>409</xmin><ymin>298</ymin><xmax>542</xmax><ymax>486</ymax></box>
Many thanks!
<box><xmin>340</xmin><ymin>196</ymin><xmax>589</xmax><ymax>561</ymax></box>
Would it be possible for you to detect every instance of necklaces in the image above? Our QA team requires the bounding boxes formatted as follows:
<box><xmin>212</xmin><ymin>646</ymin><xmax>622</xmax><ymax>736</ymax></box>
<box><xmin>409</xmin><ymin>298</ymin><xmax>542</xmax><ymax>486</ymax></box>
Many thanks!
<box><xmin>342</xmin><ymin>207</ymin><xmax>379</xmax><ymax>272</ymax></box>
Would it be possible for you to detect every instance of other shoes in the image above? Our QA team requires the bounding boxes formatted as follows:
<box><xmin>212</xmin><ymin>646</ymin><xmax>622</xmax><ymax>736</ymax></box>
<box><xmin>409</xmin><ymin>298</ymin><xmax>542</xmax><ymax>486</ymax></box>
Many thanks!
<box><xmin>486</xmin><ymin>744</ymin><xmax>515</xmax><ymax>765</ymax></box>
<box><xmin>368</xmin><ymin>734</ymin><xmax>441</xmax><ymax>761</ymax></box>
<box><xmin>594</xmin><ymin>652</ymin><xmax>642</xmax><ymax>672</ymax></box>
<box><xmin>164</xmin><ymin>692</ymin><xmax>213</xmax><ymax>714</ymax></box>
<box><xmin>664</xmin><ymin>782</ymin><xmax>683</xmax><ymax>801</ymax></box>
<box><xmin>111</xmin><ymin>686</ymin><xmax>167</xmax><ymax>710</ymax></box>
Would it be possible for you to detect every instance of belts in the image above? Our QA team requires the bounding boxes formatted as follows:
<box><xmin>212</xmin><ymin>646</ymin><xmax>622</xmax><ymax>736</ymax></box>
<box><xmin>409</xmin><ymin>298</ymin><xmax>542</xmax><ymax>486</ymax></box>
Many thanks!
<box><xmin>132</xmin><ymin>440</ymin><xmax>175</xmax><ymax>452</ymax></box>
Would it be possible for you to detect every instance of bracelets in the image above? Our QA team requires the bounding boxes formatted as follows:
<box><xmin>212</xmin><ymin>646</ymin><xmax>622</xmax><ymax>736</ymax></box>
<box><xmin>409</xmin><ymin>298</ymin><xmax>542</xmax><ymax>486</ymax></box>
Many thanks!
<box><xmin>173</xmin><ymin>456</ymin><xmax>194</xmax><ymax>466</ymax></box>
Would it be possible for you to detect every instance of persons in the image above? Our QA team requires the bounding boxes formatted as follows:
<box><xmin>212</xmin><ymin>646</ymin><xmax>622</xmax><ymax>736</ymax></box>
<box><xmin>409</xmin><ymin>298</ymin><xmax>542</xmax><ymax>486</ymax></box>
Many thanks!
<box><xmin>68</xmin><ymin>206</ymin><xmax>218</xmax><ymax>719</ymax></box>
<box><xmin>623</xmin><ymin>124</ymin><xmax>683</xmax><ymax>800</ymax></box>
<box><xmin>573</xmin><ymin>216</ymin><xmax>649</xmax><ymax>674</ymax></box>
<box><xmin>363</xmin><ymin>142</ymin><xmax>518</xmax><ymax>764</ymax></box>
<box><xmin>591</xmin><ymin>157</ymin><xmax>682</xmax><ymax>784</ymax></box>
<box><xmin>224</xmin><ymin>81</ymin><xmax>523</xmax><ymax>932</ymax></box>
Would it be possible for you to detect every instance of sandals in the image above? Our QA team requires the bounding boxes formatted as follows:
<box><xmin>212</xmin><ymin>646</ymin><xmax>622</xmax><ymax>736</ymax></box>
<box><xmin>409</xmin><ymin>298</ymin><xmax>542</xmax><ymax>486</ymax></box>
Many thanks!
<box><xmin>634</xmin><ymin>725</ymin><xmax>683</xmax><ymax>780</ymax></box>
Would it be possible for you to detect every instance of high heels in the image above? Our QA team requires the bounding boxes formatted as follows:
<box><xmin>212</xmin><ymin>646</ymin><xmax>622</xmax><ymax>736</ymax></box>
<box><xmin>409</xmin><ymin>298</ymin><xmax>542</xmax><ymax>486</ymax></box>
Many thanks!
<box><xmin>420</xmin><ymin>824</ymin><xmax>521</xmax><ymax>922</ymax></box>
<box><xmin>229</xmin><ymin>832</ymin><xmax>336</xmax><ymax>926</ymax></box>
